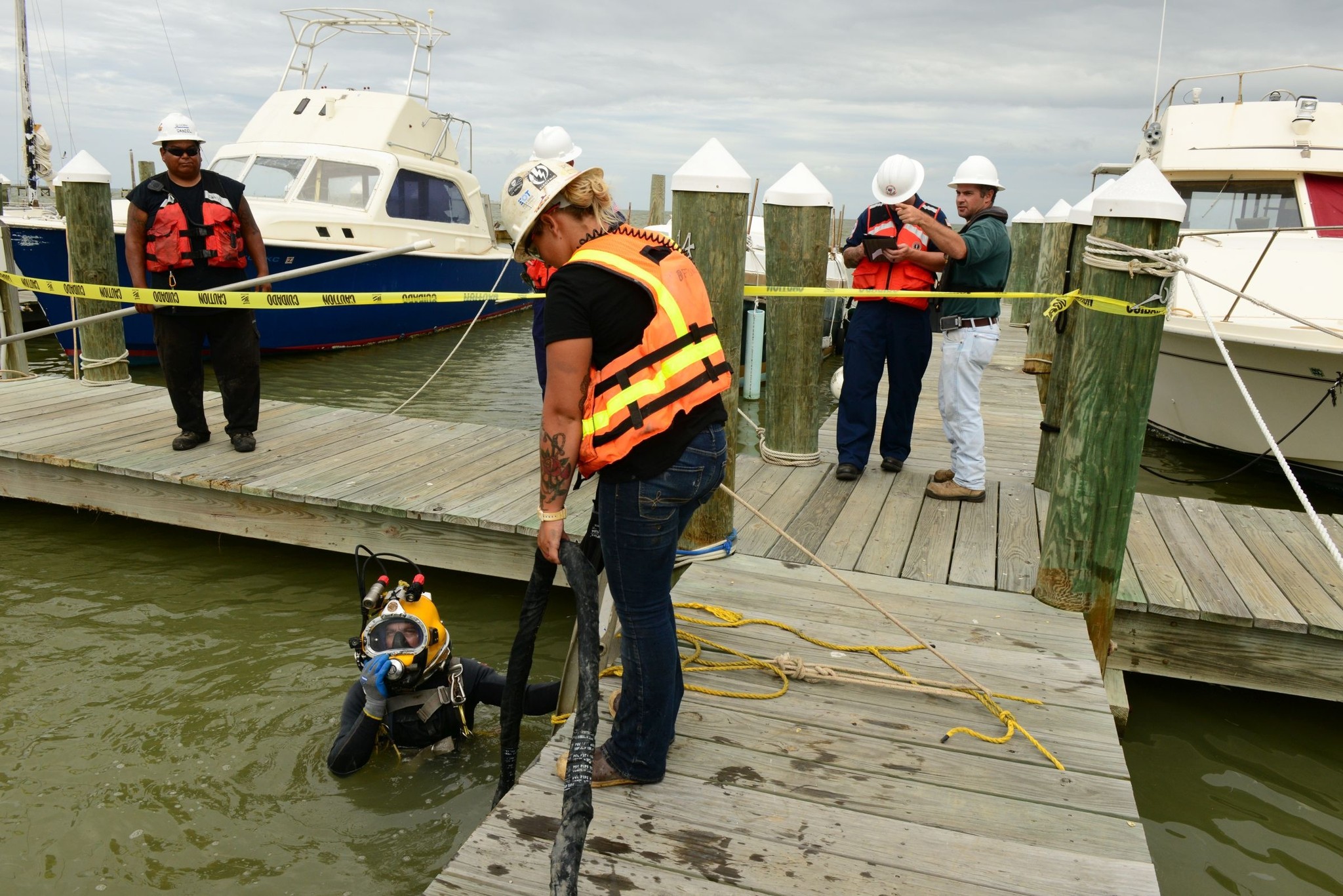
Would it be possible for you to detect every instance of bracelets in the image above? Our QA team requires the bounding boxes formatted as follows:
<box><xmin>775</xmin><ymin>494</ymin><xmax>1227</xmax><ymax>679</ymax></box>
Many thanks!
<box><xmin>536</xmin><ymin>504</ymin><xmax>567</xmax><ymax>522</ymax></box>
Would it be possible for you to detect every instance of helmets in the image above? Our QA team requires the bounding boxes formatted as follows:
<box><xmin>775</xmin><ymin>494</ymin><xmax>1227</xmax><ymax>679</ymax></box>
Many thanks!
<box><xmin>348</xmin><ymin>574</ymin><xmax>452</xmax><ymax>692</ymax></box>
<box><xmin>529</xmin><ymin>126</ymin><xmax>582</xmax><ymax>162</ymax></box>
<box><xmin>499</xmin><ymin>160</ymin><xmax>604</xmax><ymax>264</ymax></box>
<box><xmin>871</xmin><ymin>154</ymin><xmax>924</xmax><ymax>204</ymax></box>
<box><xmin>947</xmin><ymin>156</ymin><xmax>1006</xmax><ymax>191</ymax></box>
<box><xmin>152</xmin><ymin>113</ymin><xmax>206</xmax><ymax>145</ymax></box>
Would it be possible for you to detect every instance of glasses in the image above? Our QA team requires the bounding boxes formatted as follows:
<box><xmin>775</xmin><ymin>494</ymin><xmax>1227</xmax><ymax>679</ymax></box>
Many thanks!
<box><xmin>163</xmin><ymin>145</ymin><xmax>199</xmax><ymax>157</ymax></box>
<box><xmin>525</xmin><ymin>201</ymin><xmax>561</xmax><ymax>263</ymax></box>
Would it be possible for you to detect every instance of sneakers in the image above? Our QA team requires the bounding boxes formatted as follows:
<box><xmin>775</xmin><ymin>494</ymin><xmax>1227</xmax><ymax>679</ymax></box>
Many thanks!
<box><xmin>933</xmin><ymin>469</ymin><xmax>955</xmax><ymax>482</ymax></box>
<box><xmin>231</xmin><ymin>432</ymin><xmax>257</xmax><ymax>452</ymax></box>
<box><xmin>925</xmin><ymin>479</ymin><xmax>986</xmax><ymax>503</ymax></box>
<box><xmin>172</xmin><ymin>430</ymin><xmax>211</xmax><ymax>451</ymax></box>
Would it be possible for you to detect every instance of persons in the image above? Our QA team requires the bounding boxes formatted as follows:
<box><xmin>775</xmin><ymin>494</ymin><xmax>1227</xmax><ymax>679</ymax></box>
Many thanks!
<box><xmin>328</xmin><ymin>593</ymin><xmax>564</xmax><ymax>778</ymax></box>
<box><xmin>894</xmin><ymin>156</ymin><xmax>1013</xmax><ymax>502</ymax></box>
<box><xmin>501</xmin><ymin>159</ymin><xmax>733</xmax><ymax>789</ymax></box>
<box><xmin>124</xmin><ymin>112</ymin><xmax>271</xmax><ymax>453</ymax></box>
<box><xmin>836</xmin><ymin>155</ymin><xmax>953</xmax><ymax>479</ymax></box>
<box><xmin>519</xmin><ymin>126</ymin><xmax>583</xmax><ymax>403</ymax></box>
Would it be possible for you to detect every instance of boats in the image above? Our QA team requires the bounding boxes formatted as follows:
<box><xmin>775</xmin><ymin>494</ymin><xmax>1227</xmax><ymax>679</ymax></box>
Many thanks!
<box><xmin>0</xmin><ymin>4</ymin><xmax>536</xmax><ymax>369</ymax></box>
<box><xmin>1092</xmin><ymin>0</ymin><xmax>1343</xmax><ymax>499</ymax></box>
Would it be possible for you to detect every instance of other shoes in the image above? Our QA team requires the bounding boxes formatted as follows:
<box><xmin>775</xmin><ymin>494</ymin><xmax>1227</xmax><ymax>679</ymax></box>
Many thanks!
<box><xmin>836</xmin><ymin>463</ymin><xmax>860</xmax><ymax>480</ymax></box>
<box><xmin>881</xmin><ymin>456</ymin><xmax>903</xmax><ymax>472</ymax></box>
<box><xmin>609</xmin><ymin>689</ymin><xmax>622</xmax><ymax>717</ymax></box>
<box><xmin>556</xmin><ymin>746</ymin><xmax>638</xmax><ymax>787</ymax></box>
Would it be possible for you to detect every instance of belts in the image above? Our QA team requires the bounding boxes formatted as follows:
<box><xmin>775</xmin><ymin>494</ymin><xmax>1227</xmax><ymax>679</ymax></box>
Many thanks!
<box><xmin>961</xmin><ymin>317</ymin><xmax>999</xmax><ymax>328</ymax></box>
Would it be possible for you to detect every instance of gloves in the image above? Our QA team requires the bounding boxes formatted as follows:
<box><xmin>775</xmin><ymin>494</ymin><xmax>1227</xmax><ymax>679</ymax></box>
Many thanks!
<box><xmin>359</xmin><ymin>653</ymin><xmax>392</xmax><ymax>721</ymax></box>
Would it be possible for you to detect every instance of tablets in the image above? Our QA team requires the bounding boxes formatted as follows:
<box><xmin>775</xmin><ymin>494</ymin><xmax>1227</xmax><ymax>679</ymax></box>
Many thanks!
<box><xmin>862</xmin><ymin>233</ymin><xmax>897</xmax><ymax>257</ymax></box>
